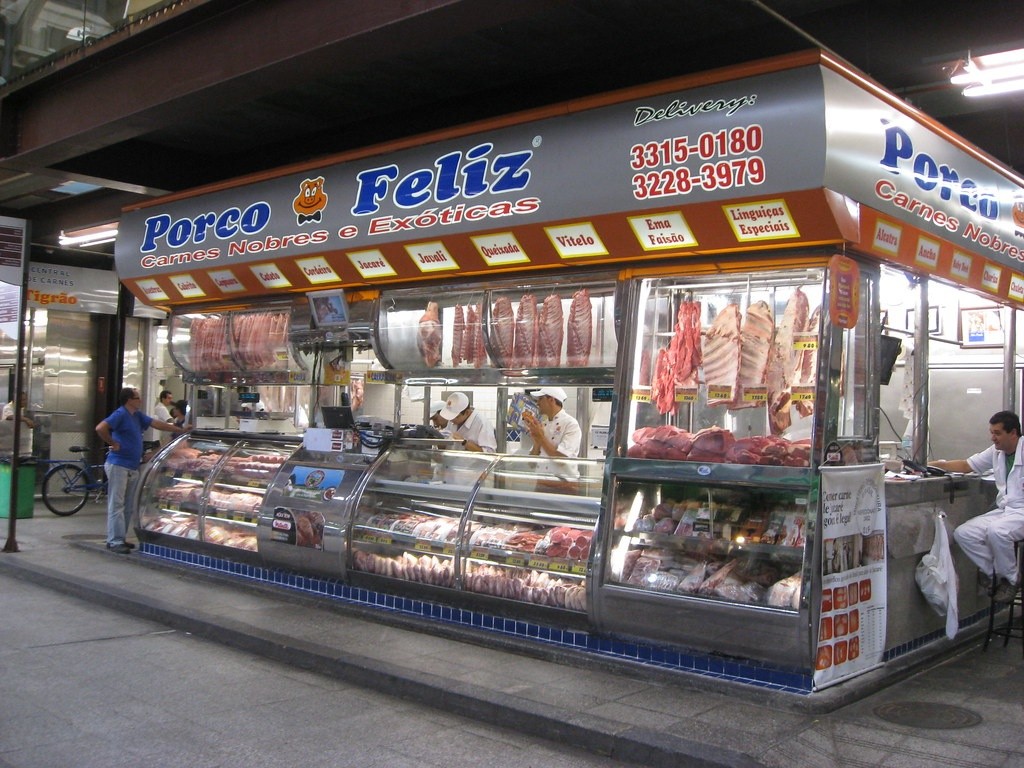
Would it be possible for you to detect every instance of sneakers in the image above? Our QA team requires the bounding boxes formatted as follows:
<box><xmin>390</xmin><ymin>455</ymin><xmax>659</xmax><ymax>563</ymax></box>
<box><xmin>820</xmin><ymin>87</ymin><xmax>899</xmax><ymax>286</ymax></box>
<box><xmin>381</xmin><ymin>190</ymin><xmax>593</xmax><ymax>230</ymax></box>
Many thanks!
<box><xmin>106</xmin><ymin>540</ymin><xmax>135</xmax><ymax>554</ymax></box>
<box><xmin>993</xmin><ymin>573</ymin><xmax>1021</xmax><ymax>603</ymax></box>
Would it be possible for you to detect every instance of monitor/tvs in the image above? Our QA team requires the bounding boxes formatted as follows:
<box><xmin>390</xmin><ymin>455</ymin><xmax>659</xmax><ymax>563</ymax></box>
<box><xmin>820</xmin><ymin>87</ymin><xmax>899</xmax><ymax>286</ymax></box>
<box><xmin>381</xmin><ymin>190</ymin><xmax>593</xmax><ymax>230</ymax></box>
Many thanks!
<box><xmin>309</xmin><ymin>294</ymin><xmax>350</xmax><ymax>327</ymax></box>
<box><xmin>880</xmin><ymin>335</ymin><xmax>902</xmax><ymax>385</ymax></box>
<box><xmin>322</xmin><ymin>406</ymin><xmax>355</xmax><ymax>430</ymax></box>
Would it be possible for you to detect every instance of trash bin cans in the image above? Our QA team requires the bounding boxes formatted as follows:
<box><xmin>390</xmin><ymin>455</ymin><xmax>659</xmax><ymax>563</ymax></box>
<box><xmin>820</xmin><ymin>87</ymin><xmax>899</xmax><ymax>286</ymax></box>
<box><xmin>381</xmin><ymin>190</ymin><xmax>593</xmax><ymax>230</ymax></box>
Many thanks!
<box><xmin>0</xmin><ymin>455</ymin><xmax>39</xmax><ymax>520</ymax></box>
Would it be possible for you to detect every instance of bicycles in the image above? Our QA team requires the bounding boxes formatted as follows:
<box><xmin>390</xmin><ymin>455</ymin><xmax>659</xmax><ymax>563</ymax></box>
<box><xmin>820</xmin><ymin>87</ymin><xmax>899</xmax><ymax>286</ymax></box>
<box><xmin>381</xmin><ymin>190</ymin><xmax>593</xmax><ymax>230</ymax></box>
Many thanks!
<box><xmin>42</xmin><ymin>446</ymin><xmax>152</xmax><ymax>516</ymax></box>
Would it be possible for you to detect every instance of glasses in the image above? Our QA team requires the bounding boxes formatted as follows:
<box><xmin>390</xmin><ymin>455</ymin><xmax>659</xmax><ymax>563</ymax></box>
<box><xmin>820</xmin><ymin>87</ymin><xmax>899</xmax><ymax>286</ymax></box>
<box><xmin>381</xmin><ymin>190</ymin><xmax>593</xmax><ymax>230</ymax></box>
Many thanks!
<box><xmin>130</xmin><ymin>396</ymin><xmax>141</xmax><ymax>400</ymax></box>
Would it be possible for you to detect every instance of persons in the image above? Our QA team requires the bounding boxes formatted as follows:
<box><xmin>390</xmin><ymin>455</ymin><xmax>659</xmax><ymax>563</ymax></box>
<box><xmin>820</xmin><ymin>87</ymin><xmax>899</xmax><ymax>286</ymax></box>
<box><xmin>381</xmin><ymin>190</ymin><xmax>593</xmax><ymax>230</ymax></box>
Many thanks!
<box><xmin>170</xmin><ymin>400</ymin><xmax>188</xmax><ymax>442</ymax></box>
<box><xmin>927</xmin><ymin>411</ymin><xmax>1024</xmax><ymax>603</ymax></box>
<box><xmin>157</xmin><ymin>380</ymin><xmax>166</xmax><ymax>397</ymax></box>
<box><xmin>96</xmin><ymin>388</ymin><xmax>192</xmax><ymax>554</ymax></box>
<box><xmin>154</xmin><ymin>390</ymin><xmax>174</xmax><ymax>446</ymax></box>
<box><xmin>237</xmin><ymin>386</ymin><xmax>266</xmax><ymax>411</ymax></box>
<box><xmin>2</xmin><ymin>391</ymin><xmax>34</xmax><ymax>428</ymax></box>
<box><xmin>524</xmin><ymin>386</ymin><xmax>582</xmax><ymax>495</ymax></box>
<box><xmin>429</xmin><ymin>392</ymin><xmax>497</xmax><ymax>487</ymax></box>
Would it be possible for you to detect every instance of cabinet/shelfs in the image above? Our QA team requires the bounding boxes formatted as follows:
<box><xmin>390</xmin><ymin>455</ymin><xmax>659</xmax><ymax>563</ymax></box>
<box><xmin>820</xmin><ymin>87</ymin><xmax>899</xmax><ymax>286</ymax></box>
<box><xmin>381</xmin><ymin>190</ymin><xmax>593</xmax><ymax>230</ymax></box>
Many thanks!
<box><xmin>130</xmin><ymin>428</ymin><xmax>609</xmax><ymax>634</ymax></box>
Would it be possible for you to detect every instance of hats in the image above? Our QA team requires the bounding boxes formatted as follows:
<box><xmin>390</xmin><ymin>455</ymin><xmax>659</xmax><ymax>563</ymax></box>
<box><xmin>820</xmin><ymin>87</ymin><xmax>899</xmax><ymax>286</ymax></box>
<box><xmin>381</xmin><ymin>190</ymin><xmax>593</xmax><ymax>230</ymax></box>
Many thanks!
<box><xmin>439</xmin><ymin>392</ymin><xmax>468</xmax><ymax>421</ymax></box>
<box><xmin>530</xmin><ymin>387</ymin><xmax>567</xmax><ymax>404</ymax></box>
<box><xmin>427</xmin><ymin>400</ymin><xmax>446</xmax><ymax>418</ymax></box>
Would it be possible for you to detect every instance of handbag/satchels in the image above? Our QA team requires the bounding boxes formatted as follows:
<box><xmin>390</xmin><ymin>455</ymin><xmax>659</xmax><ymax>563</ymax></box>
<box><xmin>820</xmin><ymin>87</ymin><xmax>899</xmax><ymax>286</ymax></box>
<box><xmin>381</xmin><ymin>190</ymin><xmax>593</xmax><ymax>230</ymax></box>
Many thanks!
<box><xmin>916</xmin><ymin>512</ymin><xmax>959</xmax><ymax>617</ymax></box>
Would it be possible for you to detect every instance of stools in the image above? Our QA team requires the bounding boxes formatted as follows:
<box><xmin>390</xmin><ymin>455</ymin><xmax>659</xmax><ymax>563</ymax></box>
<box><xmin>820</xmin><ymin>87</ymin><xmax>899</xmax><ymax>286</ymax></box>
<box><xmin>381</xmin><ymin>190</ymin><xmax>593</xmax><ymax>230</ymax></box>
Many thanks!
<box><xmin>981</xmin><ymin>538</ymin><xmax>1024</xmax><ymax>660</ymax></box>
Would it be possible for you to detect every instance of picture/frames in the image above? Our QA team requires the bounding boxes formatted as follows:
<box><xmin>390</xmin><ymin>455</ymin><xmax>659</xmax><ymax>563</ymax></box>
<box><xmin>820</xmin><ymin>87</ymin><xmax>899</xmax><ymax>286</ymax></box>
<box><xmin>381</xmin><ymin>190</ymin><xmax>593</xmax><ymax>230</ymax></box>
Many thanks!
<box><xmin>905</xmin><ymin>305</ymin><xmax>942</xmax><ymax>338</ymax></box>
<box><xmin>959</xmin><ymin>305</ymin><xmax>1004</xmax><ymax>349</ymax></box>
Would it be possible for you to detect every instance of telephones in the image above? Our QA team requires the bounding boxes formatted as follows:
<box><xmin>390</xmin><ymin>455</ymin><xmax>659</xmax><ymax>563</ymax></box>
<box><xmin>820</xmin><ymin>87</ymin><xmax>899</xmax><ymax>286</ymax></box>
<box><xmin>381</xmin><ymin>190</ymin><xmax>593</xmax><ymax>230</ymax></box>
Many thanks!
<box><xmin>902</xmin><ymin>458</ymin><xmax>928</xmax><ymax>477</ymax></box>
<box><xmin>924</xmin><ymin>464</ymin><xmax>947</xmax><ymax>478</ymax></box>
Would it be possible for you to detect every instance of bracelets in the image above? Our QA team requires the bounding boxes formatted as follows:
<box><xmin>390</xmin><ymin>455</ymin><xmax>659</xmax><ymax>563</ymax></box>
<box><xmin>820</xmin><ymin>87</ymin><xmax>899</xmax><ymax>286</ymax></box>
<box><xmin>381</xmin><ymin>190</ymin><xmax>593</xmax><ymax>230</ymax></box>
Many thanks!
<box><xmin>461</xmin><ymin>438</ymin><xmax>468</xmax><ymax>446</ymax></box>
